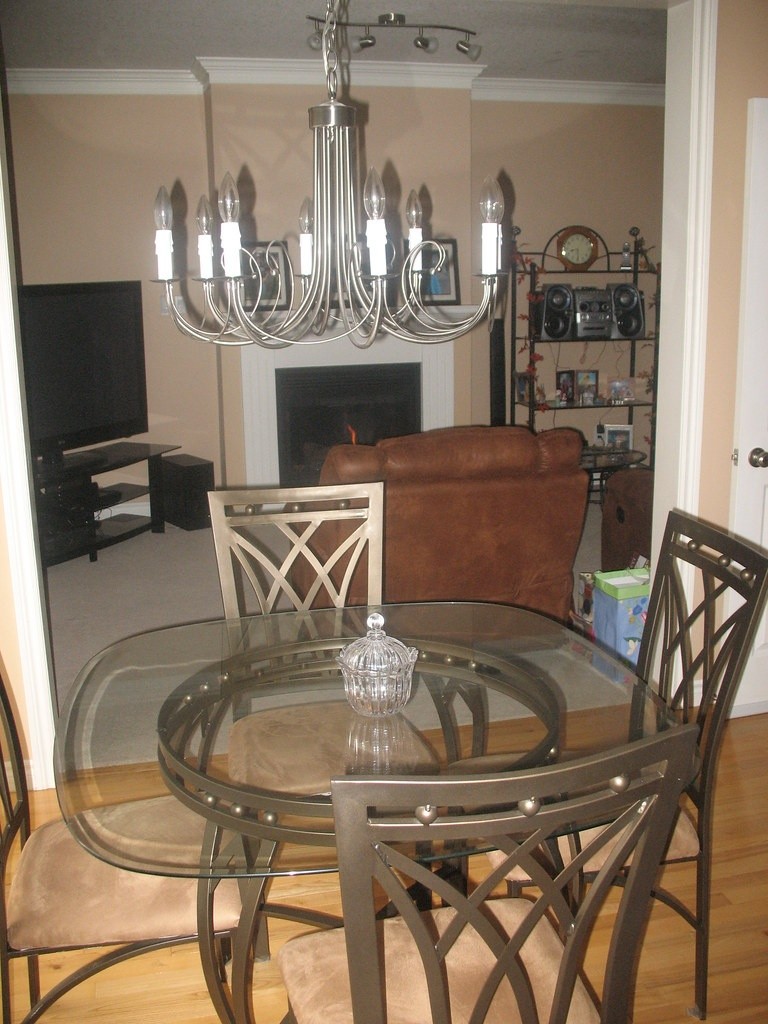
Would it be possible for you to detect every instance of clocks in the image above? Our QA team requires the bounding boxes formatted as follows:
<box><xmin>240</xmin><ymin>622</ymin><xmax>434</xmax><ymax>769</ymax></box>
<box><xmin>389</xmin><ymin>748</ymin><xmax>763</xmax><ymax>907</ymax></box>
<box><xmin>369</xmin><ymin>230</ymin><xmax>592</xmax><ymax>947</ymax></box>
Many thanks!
<box><xmin>556</xmin><ymin>226</ymin><xmax>598</xmax><ymax>273</ymax></box>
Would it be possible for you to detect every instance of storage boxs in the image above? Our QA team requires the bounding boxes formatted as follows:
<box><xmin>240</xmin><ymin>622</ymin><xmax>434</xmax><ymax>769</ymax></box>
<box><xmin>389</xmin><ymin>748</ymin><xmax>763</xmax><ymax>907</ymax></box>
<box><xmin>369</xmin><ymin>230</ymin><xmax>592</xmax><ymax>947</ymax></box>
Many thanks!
<box><xmin>594</xmin><ymin>568</ymin><xmax>650</xmax><ymax>600</ymax></box>
<box><xmin>592</xmin><ymin>585</ymin><xmax>650</xmax><ymax>684</ymax></box>
<box><xmin>578</xmin><ymin>571</ymin><xmax>595</xmax><ymax>622</ymax></box>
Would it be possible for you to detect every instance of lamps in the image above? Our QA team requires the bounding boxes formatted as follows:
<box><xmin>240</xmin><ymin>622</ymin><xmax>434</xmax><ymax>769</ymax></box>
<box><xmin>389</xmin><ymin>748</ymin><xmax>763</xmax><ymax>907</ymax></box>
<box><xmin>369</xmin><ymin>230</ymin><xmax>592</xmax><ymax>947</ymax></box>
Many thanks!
<box><xmin>153</xmin><ymin>0</ymin><xmax>505</xmax><ymax>350</ymax></box>
<box><xmin>305</xmin><ymin>12</ymin><xmax>481</xmax><ymax>61</ymax></box>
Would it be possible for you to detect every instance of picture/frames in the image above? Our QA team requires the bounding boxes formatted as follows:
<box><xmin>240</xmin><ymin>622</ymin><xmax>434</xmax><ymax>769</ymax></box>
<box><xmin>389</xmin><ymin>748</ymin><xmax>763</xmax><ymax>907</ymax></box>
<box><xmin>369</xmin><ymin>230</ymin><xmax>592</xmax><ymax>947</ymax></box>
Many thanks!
<box><xmin>516</xmin><ymin>373</ymin><xmax>531</xmax><ymax>403</ymax></box>
<box><xmin>607</xmin><ymin>377</ymin><xmax>635</xmax><ymax>399</ymax></box>
<box><xmin>604</xmin><ymin>424</ymin><xmax>634</xmax><ymax>451</ymax></box>
<box><xmin>556</xmin><ymin>370</ymin><xmax>575</xmax><ymax>402</ymax></box>
<box><xmin>404</xmin><ymin>238</ymin><xmax>461</xmax><ymax>306</ymax></box>
<box><xmin>239</xmin><ymin>241</ymin><xmax>292</xmax><ymax>312</ymax></box>
<box><xmin>575</xmin><ymin>370</ymin><xmax>599</xmax><ymax>400</ymax></box>
<box><xmin>312</xmin><ymin>233</ymin><xmax>394</xmax><ymax>308</ymax></box>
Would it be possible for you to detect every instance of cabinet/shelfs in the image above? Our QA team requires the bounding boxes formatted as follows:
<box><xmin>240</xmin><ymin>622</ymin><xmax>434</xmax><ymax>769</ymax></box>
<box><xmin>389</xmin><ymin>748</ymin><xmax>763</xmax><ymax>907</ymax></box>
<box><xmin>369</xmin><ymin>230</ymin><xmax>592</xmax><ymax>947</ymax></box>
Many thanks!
<box><xmin>511</xmin><ymin>225</ymin><xmax>661</xmax><ymax>471</ymax></box>
<box><xmin>37</xmin><ymin>442</ymin><xmax>182</xmax><ymax>567</ymax></box>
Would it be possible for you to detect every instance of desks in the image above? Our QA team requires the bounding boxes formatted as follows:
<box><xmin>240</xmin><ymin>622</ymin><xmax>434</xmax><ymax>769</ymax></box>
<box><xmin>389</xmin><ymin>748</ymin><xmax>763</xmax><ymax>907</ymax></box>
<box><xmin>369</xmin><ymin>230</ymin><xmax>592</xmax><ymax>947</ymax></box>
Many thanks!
<box><xmin>54</xmin><ymin>601</ymin><xmax>705</xmax><ymax>1024</ymax></box>
<box><xmin>580</xmin><ymin>446</ymin><xmax>647</xmax><ymax>513</ymax></box>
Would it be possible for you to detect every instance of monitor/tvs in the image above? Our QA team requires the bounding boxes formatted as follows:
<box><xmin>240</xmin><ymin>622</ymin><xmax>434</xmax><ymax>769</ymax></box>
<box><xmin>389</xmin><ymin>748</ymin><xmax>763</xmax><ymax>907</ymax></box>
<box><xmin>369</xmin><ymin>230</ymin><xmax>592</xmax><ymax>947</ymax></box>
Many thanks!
<box><xmin>19</xmin><ymin>281</ymin><xmax>150</xmax><ymax>477</ymax></box>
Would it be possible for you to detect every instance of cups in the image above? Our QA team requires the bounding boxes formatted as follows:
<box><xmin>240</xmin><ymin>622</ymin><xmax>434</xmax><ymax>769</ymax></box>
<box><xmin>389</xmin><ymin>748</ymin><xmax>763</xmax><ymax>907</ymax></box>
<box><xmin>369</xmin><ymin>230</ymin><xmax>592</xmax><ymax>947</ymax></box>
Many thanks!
<box><xmin>335</xmin><ymin>613</ymin><xmax>418</xmax><ymax>718</ymax></box>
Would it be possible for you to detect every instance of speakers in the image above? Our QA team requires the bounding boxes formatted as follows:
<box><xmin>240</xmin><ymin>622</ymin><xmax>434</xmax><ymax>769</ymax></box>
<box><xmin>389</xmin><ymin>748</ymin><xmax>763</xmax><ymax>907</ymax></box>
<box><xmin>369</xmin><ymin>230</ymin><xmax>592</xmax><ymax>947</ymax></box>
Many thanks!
<box><xmin>606</xmin><ymin>282</ymin><xmax>645</xmax><ymax>340</ymax></box>
<box><xmin>533</xmin><ymin>283</ymin><xmax>576</xmax><ymax>342</ymax></box>
<box><xmin>158</xmin><ymin>454</ymin><xmax>215</xmax><ymax>530</ymax></box>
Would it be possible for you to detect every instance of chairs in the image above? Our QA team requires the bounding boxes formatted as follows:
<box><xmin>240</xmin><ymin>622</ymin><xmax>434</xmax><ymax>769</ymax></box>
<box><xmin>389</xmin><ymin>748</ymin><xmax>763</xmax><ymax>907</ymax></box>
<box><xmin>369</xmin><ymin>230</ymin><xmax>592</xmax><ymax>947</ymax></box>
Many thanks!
<box><xmin>0</xmin><ymin>483</ymin><xmax>768</xmax><ymax>1024</ymax></box>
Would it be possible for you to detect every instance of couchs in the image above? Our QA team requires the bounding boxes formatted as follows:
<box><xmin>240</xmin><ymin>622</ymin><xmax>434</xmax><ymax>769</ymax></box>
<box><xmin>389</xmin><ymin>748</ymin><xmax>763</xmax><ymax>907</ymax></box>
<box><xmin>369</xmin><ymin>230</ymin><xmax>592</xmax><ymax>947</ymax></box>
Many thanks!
<box><xmin>601</xmin><ymin>468</ymin><xmax>654</xmax><ymax>570</ymax></box>
<box><xmin>289</xmin><ymin>426</ymin><xmax>589</xmax><ymax>625</ymax></box>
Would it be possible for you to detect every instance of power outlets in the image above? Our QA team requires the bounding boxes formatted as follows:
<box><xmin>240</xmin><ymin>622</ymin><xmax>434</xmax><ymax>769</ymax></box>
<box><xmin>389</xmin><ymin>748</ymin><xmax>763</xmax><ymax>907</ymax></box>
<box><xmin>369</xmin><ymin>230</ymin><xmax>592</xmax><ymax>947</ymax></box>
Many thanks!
<box><xmin>594</xmin><ymin>426</ymin><xmax>605</xmax><ymax>446</ymax></box>
